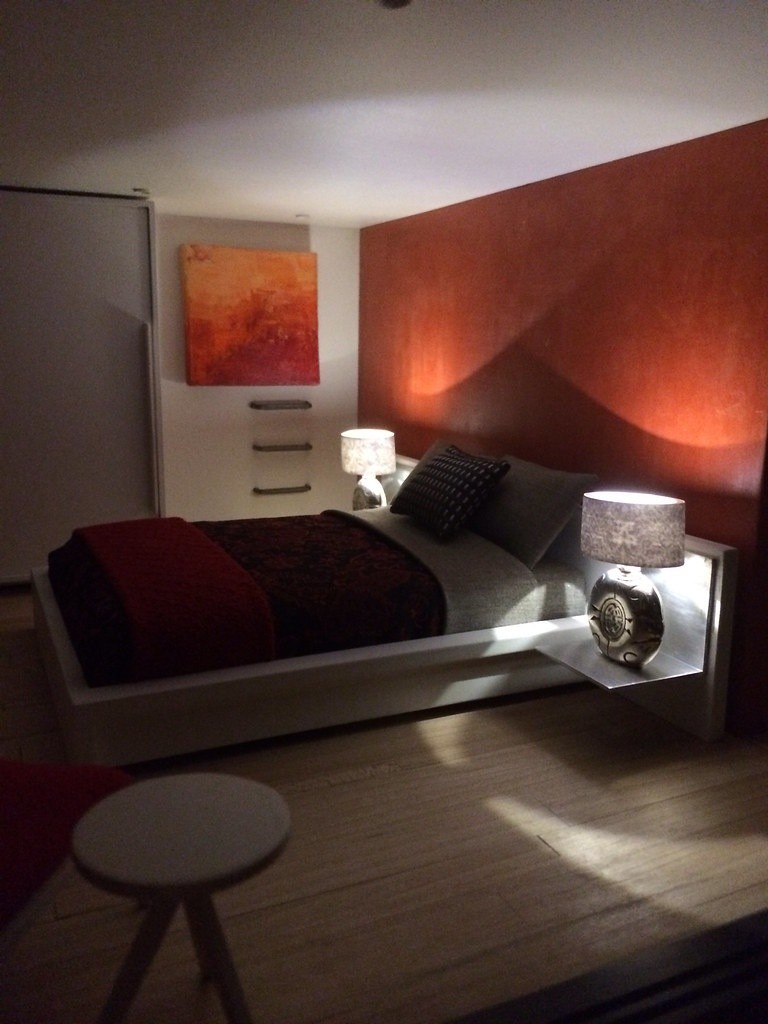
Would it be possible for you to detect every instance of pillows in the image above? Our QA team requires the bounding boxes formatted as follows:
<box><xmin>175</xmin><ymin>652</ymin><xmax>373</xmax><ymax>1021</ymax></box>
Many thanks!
<box><xmin>390</xmin><ymin>445</ymin><xmax>510</xmax><ymax>543</ymax></box>
<box><xmin>390</xmin><ymin>437</ymin><xmax>498</xmax><ymax>507</ymax></box>
<box><xmin>466</xmin><ymin>453</ymin><xmax>603</xmax><ymax>570</ymax></box>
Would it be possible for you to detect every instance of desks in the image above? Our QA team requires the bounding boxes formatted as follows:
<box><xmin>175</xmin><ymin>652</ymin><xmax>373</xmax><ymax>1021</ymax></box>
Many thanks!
<box><xmin>67</xmin><ymin>772</ymin><xmax>292</xmax><ymax>1024</ymax></box>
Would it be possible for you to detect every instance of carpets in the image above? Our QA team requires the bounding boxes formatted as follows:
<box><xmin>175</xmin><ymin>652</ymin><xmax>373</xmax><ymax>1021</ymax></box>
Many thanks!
<box><xmin>441</xmin><ymin>906</ymin><xmax>768</xmax><ymax>1024</ymax></box>
<box><xmin>0</xmin><ymin>753</ymin><xmax>137</xmax><ymax>947</ymax></box>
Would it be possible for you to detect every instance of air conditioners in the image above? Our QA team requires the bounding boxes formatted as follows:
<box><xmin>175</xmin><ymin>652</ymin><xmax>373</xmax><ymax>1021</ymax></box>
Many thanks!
<box><xmin>580</xmin><ymin>492</ymin><xmax>687</xmax><ymax>670</ymax></box>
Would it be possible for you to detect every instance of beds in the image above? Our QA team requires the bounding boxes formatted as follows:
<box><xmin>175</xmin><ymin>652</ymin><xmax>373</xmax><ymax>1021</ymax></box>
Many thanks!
<box><xmin>32</xmin><ymin>462</ymin><xmax>611</xmax><ymax>768</ymax></box>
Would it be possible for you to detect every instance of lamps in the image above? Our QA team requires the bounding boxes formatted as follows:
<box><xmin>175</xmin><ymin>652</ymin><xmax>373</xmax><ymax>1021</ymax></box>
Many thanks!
<box><xmin>341</xmin><ymin>428</ymin><xmax>397</xmax><ymax>510</ymax></box>
